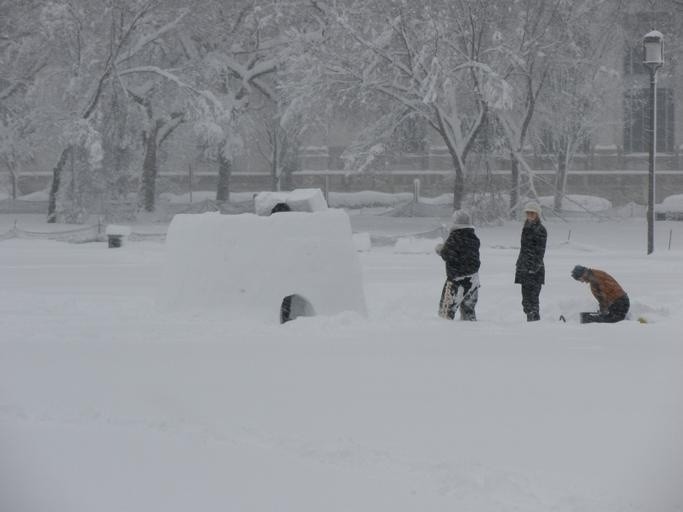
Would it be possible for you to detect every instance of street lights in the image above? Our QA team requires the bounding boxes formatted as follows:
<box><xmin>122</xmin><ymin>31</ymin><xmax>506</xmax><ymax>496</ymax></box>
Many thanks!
<box><xmin>641</xmin><ymin>30</ymin><xmax>666</xmax><ymax>255</ymax></box>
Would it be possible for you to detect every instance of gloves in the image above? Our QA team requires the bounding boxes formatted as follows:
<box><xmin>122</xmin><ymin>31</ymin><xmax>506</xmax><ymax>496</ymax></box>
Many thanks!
<box><xmin>525</xmin><ymin>272</ymin><xmax>536</xmax><ymax>289</ymax></box>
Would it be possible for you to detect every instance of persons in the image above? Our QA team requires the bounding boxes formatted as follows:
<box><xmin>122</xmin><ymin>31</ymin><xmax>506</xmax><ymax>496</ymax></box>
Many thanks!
<box><xmin>433</xmin><ymin>209</ymin><xmax>483</xmax><ymax>322</ymax></box>
<box><xmin>569</xmin><ymin>263</ymin><xmax>631</xmax><ymax>324</ymax></box>
<box><xmin>513</xmin><ymin>200</ymin><xmax>548</xmax><ymax>323</ymax></box>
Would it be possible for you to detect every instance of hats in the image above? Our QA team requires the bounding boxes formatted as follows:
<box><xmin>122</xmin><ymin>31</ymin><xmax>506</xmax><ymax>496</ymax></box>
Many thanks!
<box><xmin>524</xmin><ymin>202</ymin><xmax>539</xmax><ymax>216</ymax></box>
<box><xmin>453</xmin><ymin>211</ymin><xmax>471</xmax><ymax>225</ymax></box>
<box><xmin>572</xmin><ymin>265</ymin><xmax>589</xmax><ymax>282</ymax></box>
